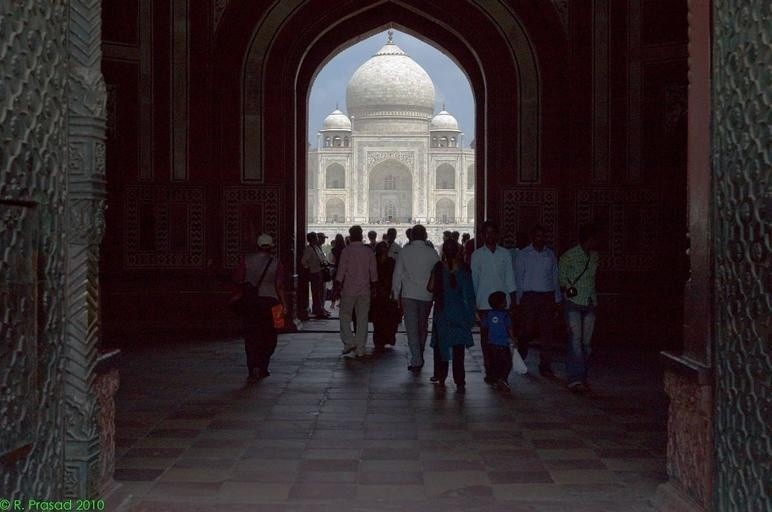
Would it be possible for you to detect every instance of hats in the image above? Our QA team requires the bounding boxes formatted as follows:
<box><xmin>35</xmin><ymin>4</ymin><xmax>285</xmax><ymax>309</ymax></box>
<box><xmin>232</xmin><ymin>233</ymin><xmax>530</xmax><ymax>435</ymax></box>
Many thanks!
<box><xmin>257</xmin><ymin>233</ymin><xmax>275</xmax><ymax>247</ymax></box>
<box><xmin>443</xmin><ymin>238</ymin><xmax>460</xmax><ymax>257</ymax></box>
<box><xmin>317</xmin><ymin>233</ymin><xmax>328</xmax><ymax>238</ymax></box>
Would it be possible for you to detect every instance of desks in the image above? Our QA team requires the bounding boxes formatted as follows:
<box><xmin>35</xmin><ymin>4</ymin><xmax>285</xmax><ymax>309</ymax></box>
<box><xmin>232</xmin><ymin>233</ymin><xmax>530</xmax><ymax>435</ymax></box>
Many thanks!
<box><xmin>95</xmin><ymin>343</ymin><xmax>121</xmax><ymax>499</ymax></box>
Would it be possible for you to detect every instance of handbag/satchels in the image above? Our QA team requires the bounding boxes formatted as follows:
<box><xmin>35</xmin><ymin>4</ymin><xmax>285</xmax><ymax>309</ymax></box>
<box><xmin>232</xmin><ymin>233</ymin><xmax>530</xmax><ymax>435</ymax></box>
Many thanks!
<box><xmin>223</xmin><ymin>282</ymin><xmax>258</xmax><ymax>314</ymax></box>
<box><xmin>324</xmin><ymin>267</ymin><xmax>334</xmax><ymax>282</ymax></box>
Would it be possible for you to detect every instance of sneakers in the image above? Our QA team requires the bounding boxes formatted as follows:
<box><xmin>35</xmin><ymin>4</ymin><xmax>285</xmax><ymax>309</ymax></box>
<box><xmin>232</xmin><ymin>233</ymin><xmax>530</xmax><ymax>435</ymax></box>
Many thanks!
<box><xmin>407</xmin><ymin>359</ymin><xmax>590</xmax><ymax>394</ymax></box>
<box><xmin>247</xmin><ymin>367</ymin><xmax>260</xmax><ymax>384</ymax></box>
<box><xmin>298</xmin><ymin>309</ymin><xmax>386</xmax><ymax>358</ymax></box>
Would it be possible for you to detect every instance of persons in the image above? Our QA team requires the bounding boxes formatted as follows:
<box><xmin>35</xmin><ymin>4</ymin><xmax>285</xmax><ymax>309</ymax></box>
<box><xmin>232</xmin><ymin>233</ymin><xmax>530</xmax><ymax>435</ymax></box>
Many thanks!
<box><xmin>235</xmin><ymin>235</ymin><xmax>287</xmax><ymax>385</ymax></box>
<box><xmin>301</xmin><ymin>225</ymin><xmax>602</xmax><ymax>395</ymax></box>
